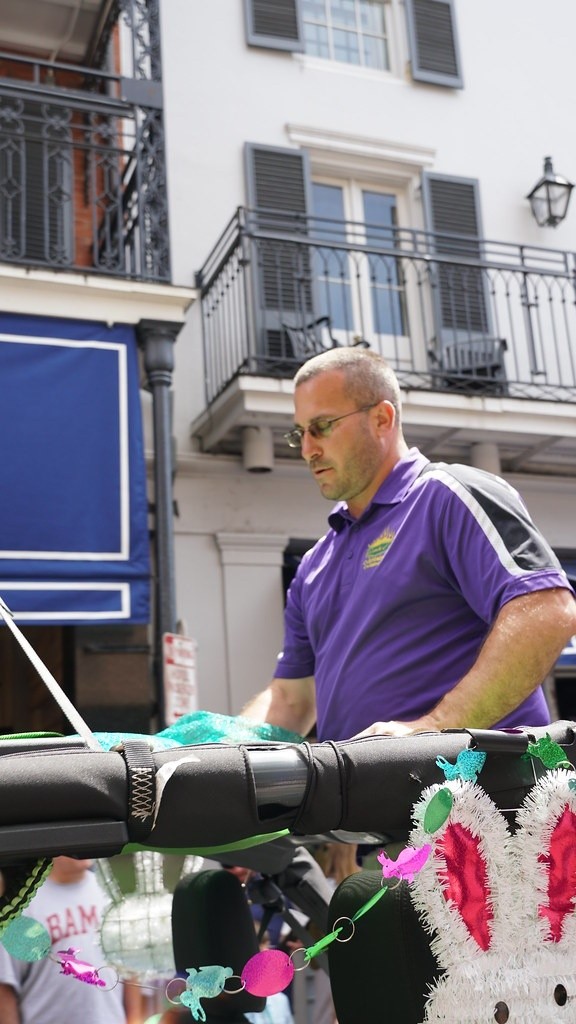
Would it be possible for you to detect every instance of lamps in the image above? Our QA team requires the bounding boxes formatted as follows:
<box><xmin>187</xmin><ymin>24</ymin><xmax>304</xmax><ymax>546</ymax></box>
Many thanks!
<box><xmin>526</xmin><ymin>157</ymin><xmax>575</xmax><ymax>229</ymax></box>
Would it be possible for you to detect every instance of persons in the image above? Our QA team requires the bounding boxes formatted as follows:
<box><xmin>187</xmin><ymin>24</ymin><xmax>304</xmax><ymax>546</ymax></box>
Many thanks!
<box><xmin>236</xmin><ymin>346</ymin><xmax>576</xmax><ymax>738</ymax></box>
<box><xmin>0</xmin><ymin>856</ymin><xmax>143</xmax><ymax>1024</ymax></box>
<box><xmin>143</xmin><ymin>841</ymin><xmax>377</xmax><ymax>1024</ymax></box>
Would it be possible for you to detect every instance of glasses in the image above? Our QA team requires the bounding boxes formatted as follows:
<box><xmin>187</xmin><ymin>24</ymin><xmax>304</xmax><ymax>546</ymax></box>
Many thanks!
<box><xmin>283</xmin><ymin>404</ymin><xmax>375</xmax><ymax>448</ymax></box>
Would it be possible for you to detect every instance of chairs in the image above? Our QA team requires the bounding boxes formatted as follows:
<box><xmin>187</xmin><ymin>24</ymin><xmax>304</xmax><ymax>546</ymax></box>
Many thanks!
<box><xmin>430</xmin><ymin>339</ymin><xmax>510</xmax><ymax>398</ymax></box>
<box><xmin>282</xmin><ymin>317</ymin><xmax>370</xmax><ymax>378</ymax></box>
